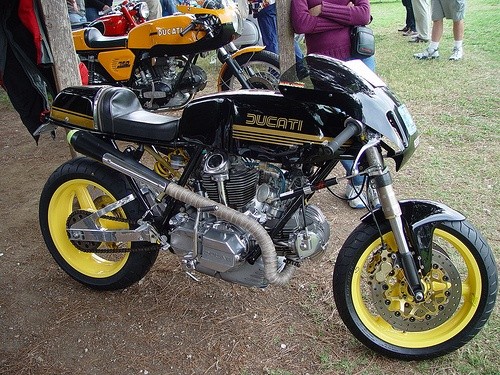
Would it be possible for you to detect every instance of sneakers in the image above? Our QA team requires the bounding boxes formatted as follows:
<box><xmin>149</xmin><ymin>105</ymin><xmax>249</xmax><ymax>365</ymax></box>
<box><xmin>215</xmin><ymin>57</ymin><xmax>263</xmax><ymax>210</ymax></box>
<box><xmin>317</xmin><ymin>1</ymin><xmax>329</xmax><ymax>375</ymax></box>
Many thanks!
<box><xmin>413</xmin><ymin>49</ymin><xmax>440</xmax><ymax>60</ymax></box>
<box><xmin>346</xmin><ymin>184</ymin><xmax>382</xmax><ymax>208</ymax></box>
<box><xmin>449</xmin><ymin>49</ymin><xmax>464</xmax><ymax>60</ymax></box>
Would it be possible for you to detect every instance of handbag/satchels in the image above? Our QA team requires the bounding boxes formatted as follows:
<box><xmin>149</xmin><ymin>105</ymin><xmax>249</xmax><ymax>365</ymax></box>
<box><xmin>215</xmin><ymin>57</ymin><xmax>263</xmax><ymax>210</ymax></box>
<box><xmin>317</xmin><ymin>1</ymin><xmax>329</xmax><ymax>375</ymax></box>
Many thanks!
<box><xmin>350</xmin><ymin>25</ymin><xmax>375</xmax><ymax>58</ymax></box>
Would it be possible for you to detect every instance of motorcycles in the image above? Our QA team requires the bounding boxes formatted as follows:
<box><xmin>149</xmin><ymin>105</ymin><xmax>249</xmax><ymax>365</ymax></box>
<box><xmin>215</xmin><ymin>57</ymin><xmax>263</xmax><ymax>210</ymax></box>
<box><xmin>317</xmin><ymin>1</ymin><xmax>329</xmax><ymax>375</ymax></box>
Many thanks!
<box><xmin>72</xmin><ymin>0</ymin><xmax>281</xmax><ymax>112</ymax></box>
<box><xmin>33</xmin><ymin>53</ymin><xmax>499</xmax><ymax>362</ymax></box>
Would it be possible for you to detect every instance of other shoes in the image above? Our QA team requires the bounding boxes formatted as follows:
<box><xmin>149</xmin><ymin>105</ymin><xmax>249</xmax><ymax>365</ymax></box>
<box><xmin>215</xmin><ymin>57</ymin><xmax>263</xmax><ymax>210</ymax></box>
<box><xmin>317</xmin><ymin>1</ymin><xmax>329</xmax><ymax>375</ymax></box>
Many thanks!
<box><xmin>403</xmin><ymin>30</ymin><xmax>418</xmax><ymax>36</ymax></box>
<box><xmin>410</xmin><ymin>35</ymin><xmax>428</xmax><ymax>42</ymax></box>
<box><xmin>398</xmin><ymin>26</ymin><xmax>409</xmax><ymax>31</ymax></box>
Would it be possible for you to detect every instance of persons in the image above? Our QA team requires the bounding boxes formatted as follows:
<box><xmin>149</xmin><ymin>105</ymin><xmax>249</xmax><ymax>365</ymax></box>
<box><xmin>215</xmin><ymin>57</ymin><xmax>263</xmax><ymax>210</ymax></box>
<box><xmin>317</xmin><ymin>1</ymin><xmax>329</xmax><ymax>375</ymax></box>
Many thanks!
<box><xmin>248</xmin><ymin>0</ymin><xmax>309</xmax><ymax>90</ymax></box>
<box><xmin>409</xmin><ymin>0</ymin><xmax>431</xmax><ymax>43</ymax></box>
<box><xmin>398</xmin><ymin>0</ymin><xmax>418</xmax><ymax>36</ymax></box>
<box><xmin>291</xmin><ymin>1</ymin><xmax>383</xmax><ymax>208</ymax></box>
<box><xmin>68</xmin><ymin>0</ymin><xmax>248</xmax><ymax>78</ymax></box>
<box><xmin>414</xmin><ymin>0</ymin><xmax>464</xmax><ymax>61</ymax></box>
